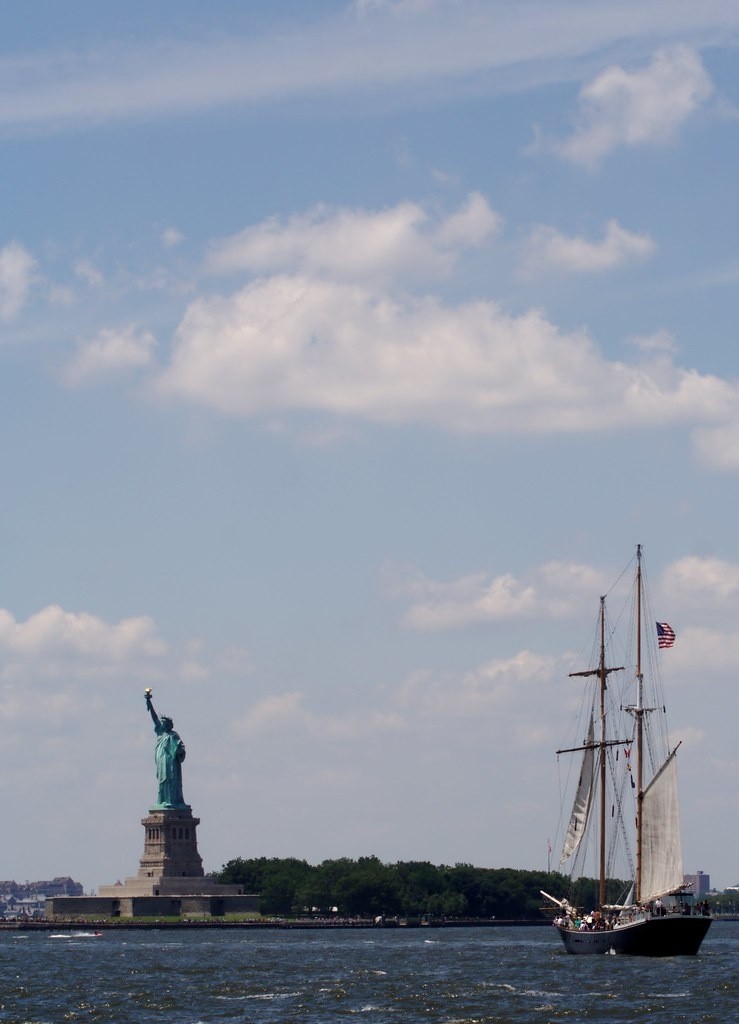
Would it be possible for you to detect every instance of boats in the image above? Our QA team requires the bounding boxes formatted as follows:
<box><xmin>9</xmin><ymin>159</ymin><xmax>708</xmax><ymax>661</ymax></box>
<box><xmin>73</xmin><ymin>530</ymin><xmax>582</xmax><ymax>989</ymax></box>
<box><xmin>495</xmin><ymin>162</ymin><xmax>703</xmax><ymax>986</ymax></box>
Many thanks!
<box><xmin>373</xmin><ymin>912</ymin><xmax>436</xmax><ymax>929</ymax></box>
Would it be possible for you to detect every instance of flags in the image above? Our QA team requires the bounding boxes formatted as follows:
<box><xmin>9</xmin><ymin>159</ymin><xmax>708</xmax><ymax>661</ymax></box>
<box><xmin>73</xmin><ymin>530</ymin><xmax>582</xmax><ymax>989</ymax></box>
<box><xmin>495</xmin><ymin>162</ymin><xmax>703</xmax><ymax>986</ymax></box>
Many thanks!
<box><xmin>655</xmin><ymin>621</ymin><xmax>676</xmax><ymax>649</ymax></box>
<box><xmin>624</xmin><ymin>748</ymin><xmax>636</xmax><ymax>788</ymax></box>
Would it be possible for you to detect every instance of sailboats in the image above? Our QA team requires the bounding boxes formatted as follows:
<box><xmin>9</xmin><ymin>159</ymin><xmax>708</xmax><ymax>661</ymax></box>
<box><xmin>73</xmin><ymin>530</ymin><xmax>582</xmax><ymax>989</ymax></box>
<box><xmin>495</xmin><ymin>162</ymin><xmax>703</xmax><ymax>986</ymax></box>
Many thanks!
<box><xmin>550</xmin><ymin>542</ymin><xmax>720</xmax><ymax>956</ymax></box>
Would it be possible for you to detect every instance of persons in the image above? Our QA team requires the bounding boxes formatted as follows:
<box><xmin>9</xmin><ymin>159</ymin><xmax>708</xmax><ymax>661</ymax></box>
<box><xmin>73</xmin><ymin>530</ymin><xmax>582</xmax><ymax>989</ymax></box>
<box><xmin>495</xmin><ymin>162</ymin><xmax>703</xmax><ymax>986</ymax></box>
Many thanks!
<box><xmin>673</xmin><ymin>907</ymin><xmax>677</xmax><ymax>912</ymax></box>
<box><xmin>635</xmin><ymin>901</ymin><xmax>653</xmax><ymax>915</ymax></box>
<box><xmin>685</xmin><ymin>903</ymin><xmax>690</xmax><ymax>915</ymax></box>
<box><xmin>295</xmin><ymin>916</ymin><xmax>352</xmax><ymax>921</ymax></box>
<box><xmin>656</xmin><ymin>898</ymin><xmax>663</xmax><ymax>915</ymax></box>
<box><xmin>146</xmin><ymin>699</ymin><xmax>186</xmax><ymax>804</ymax></box>
<box><xmin>555</xmin><ymin>909</ymin><xmax>619</xmax><ymax>932</ymax></box>
<box><xmin>0</xmin><ymin>915</ymin><xmax>288</xmax><ymax>925</ymax></box>
<box><xmin>695</xmin><ymin>900</ymin><xmax>709</xmax><ymax>915</ymax></box>
